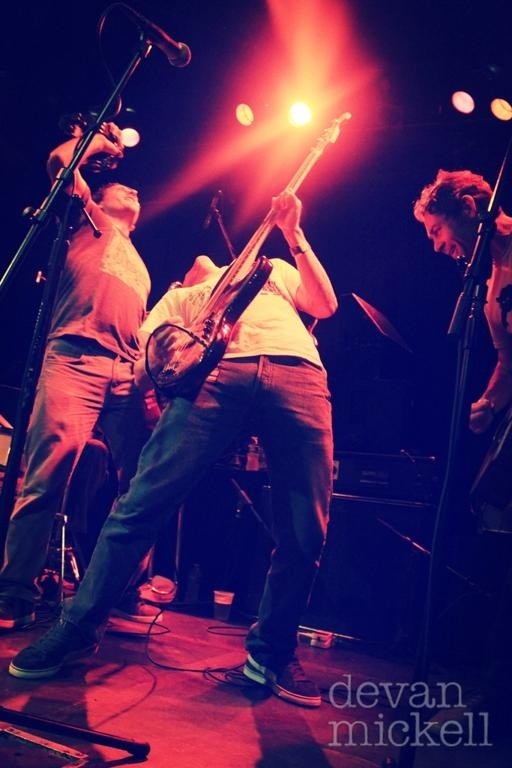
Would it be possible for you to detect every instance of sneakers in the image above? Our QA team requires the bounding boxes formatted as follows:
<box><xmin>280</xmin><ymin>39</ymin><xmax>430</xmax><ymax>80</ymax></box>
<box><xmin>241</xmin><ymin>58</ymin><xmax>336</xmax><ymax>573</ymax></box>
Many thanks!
<box><xmin>427</xmin><ymin>691</ymin><xmax>486</xmax><ymax>734</ymax></box>
<box><xmin>8</xmin><ymin>615</ymin><xmax>98</xmax><ymax>680</ymax></box>
<box><xmin>243</xmin><ymin>650</ymin><xmax>322</xmax><ymax>706</ymax></box>
<box><xmin>112</xmin><ymin>601</ymin><xmax>163</xmax><ymax>624</ymax></box>
<box><xmin>0</xmin><ymin>611</ymin><xmax>35</xmax><ymax>628</ymax></box>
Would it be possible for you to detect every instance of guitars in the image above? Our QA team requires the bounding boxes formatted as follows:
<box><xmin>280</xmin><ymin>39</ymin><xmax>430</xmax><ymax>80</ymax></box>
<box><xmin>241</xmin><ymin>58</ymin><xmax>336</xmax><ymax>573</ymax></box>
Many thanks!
<box><xmin>467</xmin><ymin>410</ymin><xmax>510</xmax><ymax>498</ymax></box>
<box><xmin>152</xmin><ymin>111</ymin><xmax>351</xmax><ymax>388</ymax></box>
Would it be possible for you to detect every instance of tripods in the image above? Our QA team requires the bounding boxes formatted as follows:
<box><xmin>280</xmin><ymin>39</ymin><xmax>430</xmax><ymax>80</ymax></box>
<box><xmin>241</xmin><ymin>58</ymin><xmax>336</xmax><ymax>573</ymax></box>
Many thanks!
<box><xmin>29</xmin><ymin>454</ymin><xmax>81</xmax><ymax>613</ymax></box>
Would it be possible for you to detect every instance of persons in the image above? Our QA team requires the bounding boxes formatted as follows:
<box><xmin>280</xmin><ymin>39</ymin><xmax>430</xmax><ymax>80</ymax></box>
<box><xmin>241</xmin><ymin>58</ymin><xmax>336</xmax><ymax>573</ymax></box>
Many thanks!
<box><xmin>414</xmin><ymin>170</ymin><xmax>512</xmax><ymax>433</ymax></box>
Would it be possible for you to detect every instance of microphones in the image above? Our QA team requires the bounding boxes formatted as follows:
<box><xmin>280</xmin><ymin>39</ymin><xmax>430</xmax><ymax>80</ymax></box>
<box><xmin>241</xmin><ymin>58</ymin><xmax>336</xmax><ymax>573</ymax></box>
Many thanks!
<box><xmin>113</xmin><ymin>0</ymin><xmax>194</xmax><ymax>68</ymax></box>
<box><xmin>201</xmin><ymin>189</ymin><xmax>227</xmax><ymax>228</ymax></box>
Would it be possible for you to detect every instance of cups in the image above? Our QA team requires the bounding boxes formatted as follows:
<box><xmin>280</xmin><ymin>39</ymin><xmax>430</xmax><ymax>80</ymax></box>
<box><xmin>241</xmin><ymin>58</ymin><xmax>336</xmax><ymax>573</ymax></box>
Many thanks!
<box><xmin>211</xmin><ymin>590</ymin><xmax>236</xmax><ymax>625</ymax></box>
